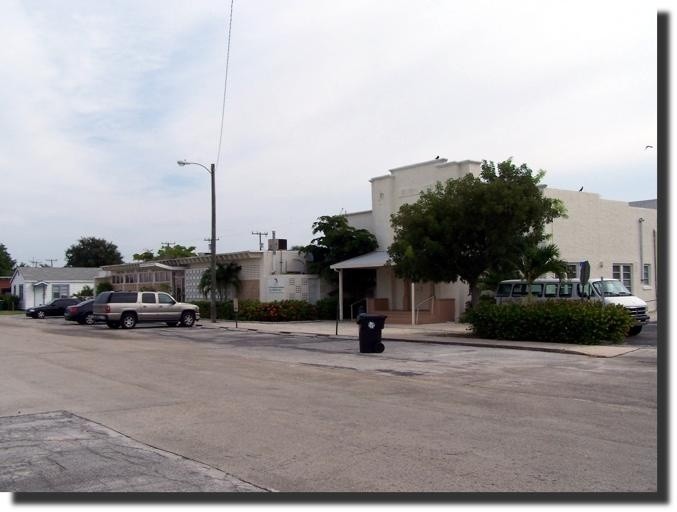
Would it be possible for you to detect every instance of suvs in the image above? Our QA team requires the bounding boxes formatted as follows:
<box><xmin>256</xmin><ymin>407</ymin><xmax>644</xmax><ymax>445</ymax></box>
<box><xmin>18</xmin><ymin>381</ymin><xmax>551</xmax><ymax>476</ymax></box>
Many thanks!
<box><xmin>92</xmin><ymin>289</ymin><xmax>201</xmax><ymax>330</ymax></box>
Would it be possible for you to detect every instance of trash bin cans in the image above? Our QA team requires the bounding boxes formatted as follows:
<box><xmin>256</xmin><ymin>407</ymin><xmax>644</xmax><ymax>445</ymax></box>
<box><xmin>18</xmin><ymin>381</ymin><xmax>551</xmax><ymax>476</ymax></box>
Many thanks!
<box><xmin>357</xmin><ymin>313</ymin><xmax>388</xmax><ymax>353</ymax></box>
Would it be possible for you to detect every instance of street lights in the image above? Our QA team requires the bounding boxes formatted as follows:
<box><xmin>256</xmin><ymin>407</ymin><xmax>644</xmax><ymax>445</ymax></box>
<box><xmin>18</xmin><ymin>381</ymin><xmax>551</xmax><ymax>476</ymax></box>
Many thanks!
<box><xmin>177</xmin><ymin>159</ymin><xmax>218</xmax><ymax>323</ymax></box>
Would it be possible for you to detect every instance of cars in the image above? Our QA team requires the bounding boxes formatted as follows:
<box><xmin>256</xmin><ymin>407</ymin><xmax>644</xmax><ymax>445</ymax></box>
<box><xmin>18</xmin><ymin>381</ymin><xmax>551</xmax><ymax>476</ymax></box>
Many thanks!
<box><xmin>64</xmin><ymin>298</ymin><xmax>107</xmax><ymax>326</ymax></box>
<box><xmin>25</xmin><ymin>297</ymin><xmax>82</xmax><ymax>320</ymax></box>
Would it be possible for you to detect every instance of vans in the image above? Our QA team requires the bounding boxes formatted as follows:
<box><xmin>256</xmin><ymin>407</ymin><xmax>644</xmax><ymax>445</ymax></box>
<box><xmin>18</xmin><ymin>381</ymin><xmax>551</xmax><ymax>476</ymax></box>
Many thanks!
<box><xmin>494</xmin><ymin>277</ymin><xmax>651</xmax><ymax>337</ymax></box>
